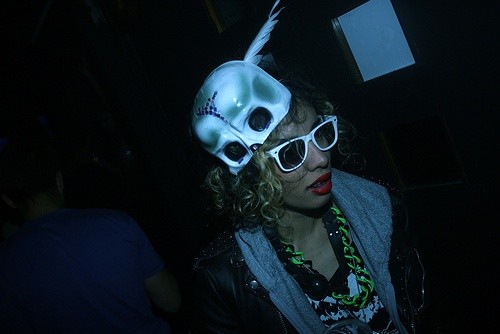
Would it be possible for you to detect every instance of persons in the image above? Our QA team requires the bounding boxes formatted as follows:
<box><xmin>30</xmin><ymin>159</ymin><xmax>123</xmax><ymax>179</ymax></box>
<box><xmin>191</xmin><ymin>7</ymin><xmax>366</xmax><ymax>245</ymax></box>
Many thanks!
<box><xmin>186</xmin><ymin>54</ymin><xmax>446</xmax><ymax>334</ymax></box>
<box><xmin>1</xmin><ymin>133</ymin><xmax>184</xmax><ymax>334</ymax></box>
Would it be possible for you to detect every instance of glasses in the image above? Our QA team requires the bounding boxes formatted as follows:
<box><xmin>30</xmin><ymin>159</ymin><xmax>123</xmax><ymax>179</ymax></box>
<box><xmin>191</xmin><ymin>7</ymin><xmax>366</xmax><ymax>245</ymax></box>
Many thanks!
<box><xmin>266</xmin><ymin>114</ymin><xmax>339</xmax><ymax>172</ymax></box>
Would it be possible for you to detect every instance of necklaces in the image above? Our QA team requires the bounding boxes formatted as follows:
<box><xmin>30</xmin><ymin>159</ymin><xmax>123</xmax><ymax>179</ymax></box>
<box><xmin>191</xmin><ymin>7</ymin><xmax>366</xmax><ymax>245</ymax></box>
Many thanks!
<box><xmin>288</xmin><ymin>206</ymin><xmax>380</xmax><ymax>334</ymax></box>
